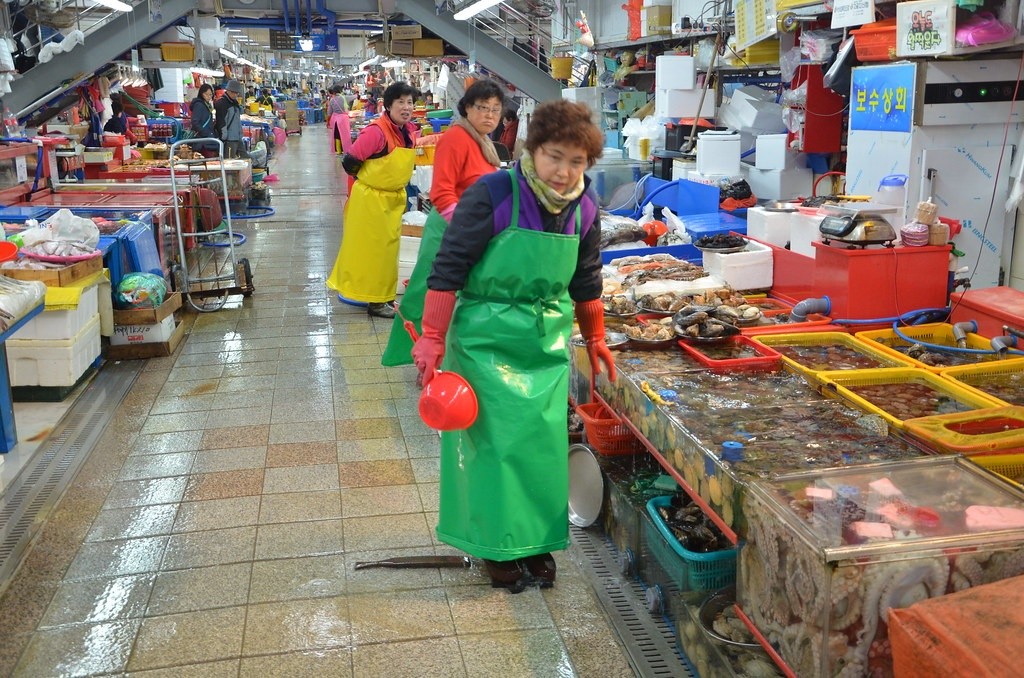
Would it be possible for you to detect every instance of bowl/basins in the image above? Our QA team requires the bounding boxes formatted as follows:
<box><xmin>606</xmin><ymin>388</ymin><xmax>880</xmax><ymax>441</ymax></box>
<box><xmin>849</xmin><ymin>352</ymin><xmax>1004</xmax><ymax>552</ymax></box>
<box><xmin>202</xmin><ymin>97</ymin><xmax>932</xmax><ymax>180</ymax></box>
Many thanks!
<box><xmin>0</xmin><ymin>242</ymin><xmax>18</xmax><ymax>262</ymax></box>
<box><xmin>696</xmin><ymin>584</ymin><xmax>779</xmax><ymax>654</ymax></box>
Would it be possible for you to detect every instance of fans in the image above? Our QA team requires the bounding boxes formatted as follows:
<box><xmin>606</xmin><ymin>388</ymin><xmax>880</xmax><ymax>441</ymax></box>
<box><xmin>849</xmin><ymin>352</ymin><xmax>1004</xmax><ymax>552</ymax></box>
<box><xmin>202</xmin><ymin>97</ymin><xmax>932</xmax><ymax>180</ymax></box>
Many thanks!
<box><xmin>24</xmin><ymin>0</ymin><xmax>76</xmax><ymax>30</ymax></box>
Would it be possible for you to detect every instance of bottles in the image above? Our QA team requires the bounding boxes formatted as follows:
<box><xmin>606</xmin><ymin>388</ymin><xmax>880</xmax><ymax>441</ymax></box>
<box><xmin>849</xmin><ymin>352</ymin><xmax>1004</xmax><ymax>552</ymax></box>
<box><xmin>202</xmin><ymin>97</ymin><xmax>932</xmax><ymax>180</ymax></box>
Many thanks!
<box><xmin>3</xmin><ymin>106</ymin><xmax>21</xmax><ymax>137</ymax></box>
<box><xmin>878</xmin><ymin>174</ymin><xmax>908</xmax><ymax>243</ymax></box>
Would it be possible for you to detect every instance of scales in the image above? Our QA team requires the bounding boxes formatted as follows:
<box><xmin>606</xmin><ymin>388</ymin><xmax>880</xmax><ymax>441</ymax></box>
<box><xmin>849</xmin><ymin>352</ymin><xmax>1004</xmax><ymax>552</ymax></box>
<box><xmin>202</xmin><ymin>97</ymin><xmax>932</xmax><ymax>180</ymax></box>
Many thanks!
<box><xmin>818</xmin><ymin>203</ymin><xmax>899</xmax><ymax>249</ymax></box>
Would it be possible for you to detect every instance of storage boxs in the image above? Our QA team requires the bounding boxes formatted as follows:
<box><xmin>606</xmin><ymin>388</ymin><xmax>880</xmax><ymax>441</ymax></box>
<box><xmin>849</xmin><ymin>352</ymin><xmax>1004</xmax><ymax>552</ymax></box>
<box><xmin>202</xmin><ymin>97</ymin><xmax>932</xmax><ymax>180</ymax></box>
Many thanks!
<box><xmin>422</xmin><ymin>125</ymin><xmax>449</xmax><ymax>137</ymax></box>
<box><xmin>187</xmin><ymin>16</ymin><xmax>221</xmax><ymax>31</ymax></box>
<box><xmin>375</xmin><ymin>42</ymin><xmax>391</xmax><ymax>55</ymax></box>
<box><xmin>391</xmin><ymin>24</ymin><xmax>422</xmax><ymax>40</ymax></box>
<box><xmin>0</xmin><ymin>83</ymin><xmax>323</xmax><ymax>404</ymax></box>
<box><xmin>390</xmin><ymin>40</ymin><xmax>413</xmax><ymax>56</ymax></box>
<box><xmin>429</xmin><ymin>117</ymin><xmax>452</xmax><ymax>132</ymax></box>
<box><xmin>141</xmin><ymin>47</ymin><xmax>163</xmax><ymax>62</ymax></box>
<box><xmin>560</xmin><ymin>0</ymin><xmax>1024</xmax><ymax>678</ymax></box>
<box><xmin>413</xmin><ymin>38</ymin><xmax>445</xmax><ymax>56</ymax></box>
<box><xmin>416</xmin><ymin>144</ymin><xmax>436</xmax><ymax>166</ymax></box>
<box><xmin>148</xmin><ymin>26</ymin><xmax>195</xmax><ymax>43</ymax></box>
<box><xmin>160</xmin><ymin>41</ymin><xmax>195</xmax><ymax>62</ymax></box>
<box><xmin>200</xmin><ymin>29</ymin><xmax>224</xmax><ymax>51</ymax></box>
<box><xmin>396</xmin><ymin>224</ymin><xmax>425</xmax><ymax>296</ymax></box>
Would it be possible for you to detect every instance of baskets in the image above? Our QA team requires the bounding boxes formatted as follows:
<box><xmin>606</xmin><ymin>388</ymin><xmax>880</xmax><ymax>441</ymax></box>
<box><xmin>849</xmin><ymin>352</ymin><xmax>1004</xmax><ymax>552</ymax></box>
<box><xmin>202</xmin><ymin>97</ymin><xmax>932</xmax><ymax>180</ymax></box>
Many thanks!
<box><xmin>752</xmin><ymin>322</ymin><xmax>1024</xmax><ymax>492</ymax></box>
<box><xmin>849</xmin><ymin>26</ymin><xmax>902</xmax><ymax>62</ymax></box>
<box><xmin>103</xmin><ymin>135</ymin><xmax>125</xmax><ymax>147</ymax></box>
<box><xmin>576</xmin><ymin>402</ymin><xmax>647</xmax><ymax>455</ymax></box>
<box><xmin>679</xmin><ymin>335</ymin><xmax>781</xmax><ymax>377</ymax></box>
<box><xmin>646</xmin><ymin>496</ymin><xmax>736</xmax><ymax>591</ymax></box>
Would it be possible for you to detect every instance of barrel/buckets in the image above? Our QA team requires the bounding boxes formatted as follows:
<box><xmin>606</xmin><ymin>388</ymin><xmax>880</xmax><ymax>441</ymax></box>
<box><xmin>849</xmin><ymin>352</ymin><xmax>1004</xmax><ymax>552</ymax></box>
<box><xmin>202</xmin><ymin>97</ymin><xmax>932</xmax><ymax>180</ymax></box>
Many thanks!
<box><xmin>550</xmin><ymin>51</ymin><xmax>575</xmax><ymax>79</ymax></box>
<box><xmin>250</xmin><ymin>103</ymin><xmax>259</xmax><ymax>115</ymax></box>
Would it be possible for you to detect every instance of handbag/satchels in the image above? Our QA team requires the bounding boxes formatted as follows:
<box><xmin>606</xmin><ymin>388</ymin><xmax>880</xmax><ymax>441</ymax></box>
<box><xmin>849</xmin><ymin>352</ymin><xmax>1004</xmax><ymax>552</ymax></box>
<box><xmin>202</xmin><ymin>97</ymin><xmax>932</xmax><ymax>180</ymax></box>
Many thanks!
<box><xmin>182</xmin><ymin>130</ymin><xmax>197</xmax><ymax>139</ymax></box>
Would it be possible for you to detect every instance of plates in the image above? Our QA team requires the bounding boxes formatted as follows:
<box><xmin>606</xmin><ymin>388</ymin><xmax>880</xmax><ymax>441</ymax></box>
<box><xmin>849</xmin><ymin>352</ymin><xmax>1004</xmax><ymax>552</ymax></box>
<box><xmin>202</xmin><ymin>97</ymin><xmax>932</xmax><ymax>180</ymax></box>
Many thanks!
<box><xmin>765</xmin><ymin>202</ymin><xmax>799</xmax><ymax>212</ymax></box>
<box><xmin>692</xmin><ymin>238</ymin><xmax>749</xmax><ymax>253</ymax></box>
<box><xmin>570</xmin><ymin>303</ymin><xmax>762</xmax><ymax>351</ymax></box>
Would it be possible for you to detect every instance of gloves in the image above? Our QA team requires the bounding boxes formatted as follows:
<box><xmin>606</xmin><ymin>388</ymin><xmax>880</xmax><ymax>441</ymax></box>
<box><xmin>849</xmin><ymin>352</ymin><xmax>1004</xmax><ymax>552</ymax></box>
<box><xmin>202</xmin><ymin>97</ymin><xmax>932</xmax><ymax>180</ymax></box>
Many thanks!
<box><xmin>575</xmin><ymin>299</ymin><xmax>616</xmax><ymax>383</ymax></box>
<box><xmin>410</xmin><ymin>290</ymin><xmax>455</xmax><ymax>387</ymax></box>
<box><xmin>124</xmin><ymin>129</ymin><xmax>137</xmax><ymax>143</ymax></box>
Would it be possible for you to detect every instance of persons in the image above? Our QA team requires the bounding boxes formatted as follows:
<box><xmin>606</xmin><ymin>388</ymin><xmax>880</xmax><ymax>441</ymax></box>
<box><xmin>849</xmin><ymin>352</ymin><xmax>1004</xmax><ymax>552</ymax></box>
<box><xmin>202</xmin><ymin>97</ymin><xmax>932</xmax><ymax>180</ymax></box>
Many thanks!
<box><xmin>413</xmin><ymin>99</ymin><xmax>617</xmax><ymax>582</ymax></box>
<box><xmin>381</xmin><ymin>81</ymin><xmax>506</xmax><ymax>388</ymax></box>
<box><xmin>103</xmin><ymin>80</ymin><xmax>518</xmax><ymax>318</ymax></box>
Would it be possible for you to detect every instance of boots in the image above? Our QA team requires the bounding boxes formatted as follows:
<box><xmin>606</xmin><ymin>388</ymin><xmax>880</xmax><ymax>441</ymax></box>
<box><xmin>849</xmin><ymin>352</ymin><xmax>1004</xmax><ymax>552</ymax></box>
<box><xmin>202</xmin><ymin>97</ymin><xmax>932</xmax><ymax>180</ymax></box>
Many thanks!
<box><xmin>335</xmin><ymin>139</ymin><xmax>341</xmax><ymax>154</ymax></box>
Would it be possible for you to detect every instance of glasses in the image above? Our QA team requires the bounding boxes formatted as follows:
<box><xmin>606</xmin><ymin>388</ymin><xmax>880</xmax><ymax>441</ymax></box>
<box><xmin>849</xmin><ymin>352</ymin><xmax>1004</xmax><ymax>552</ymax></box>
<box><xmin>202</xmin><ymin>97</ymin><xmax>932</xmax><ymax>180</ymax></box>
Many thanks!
<box><xmin>474</xmin><ymin>105</ymin><xmax>502</xmax><ymax>115</ymax></box>
<box><xmin>231</xmin><ymin>91</ymin><xmax>239</xmax><ymax>94</ymax></box>
<box><xmin>503</xmin><ymin>121</ymin><xmax>509</xmax><ymax>125</ymax></box>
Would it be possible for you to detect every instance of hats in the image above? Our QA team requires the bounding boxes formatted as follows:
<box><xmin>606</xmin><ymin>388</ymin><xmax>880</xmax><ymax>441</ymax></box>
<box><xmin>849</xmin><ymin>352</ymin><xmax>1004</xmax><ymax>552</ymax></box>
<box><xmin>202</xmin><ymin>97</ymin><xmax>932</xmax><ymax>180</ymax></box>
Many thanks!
<box><xmin>225</xmin><ymin>80</ymin><xmax>243</xmax><ymax>93</ymax></box>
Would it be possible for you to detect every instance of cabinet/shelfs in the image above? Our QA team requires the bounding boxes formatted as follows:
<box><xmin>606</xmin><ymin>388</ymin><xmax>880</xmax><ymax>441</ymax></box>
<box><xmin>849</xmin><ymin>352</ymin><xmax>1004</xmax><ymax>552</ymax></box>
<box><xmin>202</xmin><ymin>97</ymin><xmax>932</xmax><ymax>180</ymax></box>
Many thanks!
<box><xmin>586</xmin><ymin>30</ymin><xmax>726</xmax><ymax>113</ymax></box>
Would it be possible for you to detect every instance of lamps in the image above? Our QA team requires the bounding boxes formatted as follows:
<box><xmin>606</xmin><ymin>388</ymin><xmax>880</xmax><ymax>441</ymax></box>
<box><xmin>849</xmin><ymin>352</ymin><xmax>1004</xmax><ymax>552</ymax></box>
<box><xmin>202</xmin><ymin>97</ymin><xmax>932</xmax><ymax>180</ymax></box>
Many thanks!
<box><xmin>298</xmin><ymin>18</ymin><xmax>313</xmax><ymax>52</ymax></box>
<box><xmin>93</xmin><ymin>0</ymin><xmax>134</xmax><ymax>12</ymax></box>
<box><xmin>452</xmin><ymin>0</ymin><xmax>506</xmax><ymax>21</ymax></box>
<box><xmin>116</xmin><ymin>64</ymin><xmax>149</xmax><ymax>87</ymax></box>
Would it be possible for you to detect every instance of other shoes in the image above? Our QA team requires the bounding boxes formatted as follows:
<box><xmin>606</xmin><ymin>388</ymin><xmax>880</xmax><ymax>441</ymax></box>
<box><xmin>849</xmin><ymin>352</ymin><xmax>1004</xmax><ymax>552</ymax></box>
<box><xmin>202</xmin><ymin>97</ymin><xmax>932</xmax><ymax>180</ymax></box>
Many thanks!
<box><xmin>367</xmin><ymin>300</ymin><xmax>400</xmax><ymax>317</ymax></box>
<box><xmin>488</xmin><ymin>552</ymin><xmax>556</xmax><ymax>583</ymax></box>
